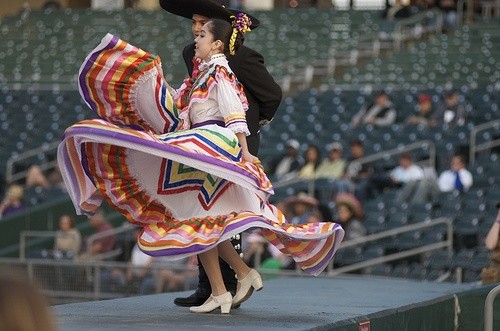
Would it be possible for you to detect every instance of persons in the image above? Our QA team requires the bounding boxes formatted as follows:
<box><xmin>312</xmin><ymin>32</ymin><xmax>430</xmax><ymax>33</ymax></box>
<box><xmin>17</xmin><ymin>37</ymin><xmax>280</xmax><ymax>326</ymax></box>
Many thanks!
<box><xmin>158</xmin><ymin>0</ymin><xmax>283</xmax><ymax>309</ymax></box>
<box><xmin>0</xmin><ymin>89</ymin><xmax>499</xmax><ymax>286</ymax></box>
<box><xmin>152</xmin><ymin>12</ymin><xmax>263</xmax><ymax>313</ymax></box>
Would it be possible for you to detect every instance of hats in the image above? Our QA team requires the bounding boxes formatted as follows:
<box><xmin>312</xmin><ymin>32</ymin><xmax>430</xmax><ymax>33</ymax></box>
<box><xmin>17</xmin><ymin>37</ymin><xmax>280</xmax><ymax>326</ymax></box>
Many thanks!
<box><xmin>158</xmin><ymin>0</ymin><xmax>260</xmax><ymax>31</ymax></box>
<box><xmin>336</xmin><ymin>192</ymin><xmax>366</xmax><ymax>219</ymax></box>
<box><xmin>283</xmin><ymin>192</ymin><xmax>318</xmax><ymax>209</ymax></box>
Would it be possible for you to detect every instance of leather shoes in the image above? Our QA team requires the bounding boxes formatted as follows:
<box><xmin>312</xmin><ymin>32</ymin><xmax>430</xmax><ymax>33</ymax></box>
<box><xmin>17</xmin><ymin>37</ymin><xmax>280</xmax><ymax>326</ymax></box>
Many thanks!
<box><xmin>174</xmin><ymin>290</ymin><xmax>204</xmax><ymax>306</ymax></box>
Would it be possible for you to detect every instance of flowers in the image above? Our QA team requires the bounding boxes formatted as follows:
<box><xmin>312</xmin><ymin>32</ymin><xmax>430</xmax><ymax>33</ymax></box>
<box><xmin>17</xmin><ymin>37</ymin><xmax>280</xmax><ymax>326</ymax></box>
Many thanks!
<box><xmin>230</xmin><ymin>12</ymin><xmax>252</xmax><ymax>33</ymax></box>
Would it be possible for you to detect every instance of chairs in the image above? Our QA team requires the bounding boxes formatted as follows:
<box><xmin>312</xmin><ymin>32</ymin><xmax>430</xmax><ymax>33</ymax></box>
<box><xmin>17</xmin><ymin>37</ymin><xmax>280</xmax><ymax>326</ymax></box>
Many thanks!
<box><xmin>0</xmin><ymin>8</ymin><xmax>500</xmax><ymax>290</ymax></box>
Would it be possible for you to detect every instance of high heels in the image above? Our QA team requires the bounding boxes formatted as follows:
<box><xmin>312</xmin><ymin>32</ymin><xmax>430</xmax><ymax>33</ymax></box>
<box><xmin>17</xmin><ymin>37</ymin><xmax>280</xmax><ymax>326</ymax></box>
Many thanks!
<box><xmin>232</xmin><ymin>269</ymin><xmax>263</xmax><ymax>307</ymax></box>
<box><xmin>190</xmin><ymin>291</ymin><xmax>233</xmax><ymax>316</ymax></box>
